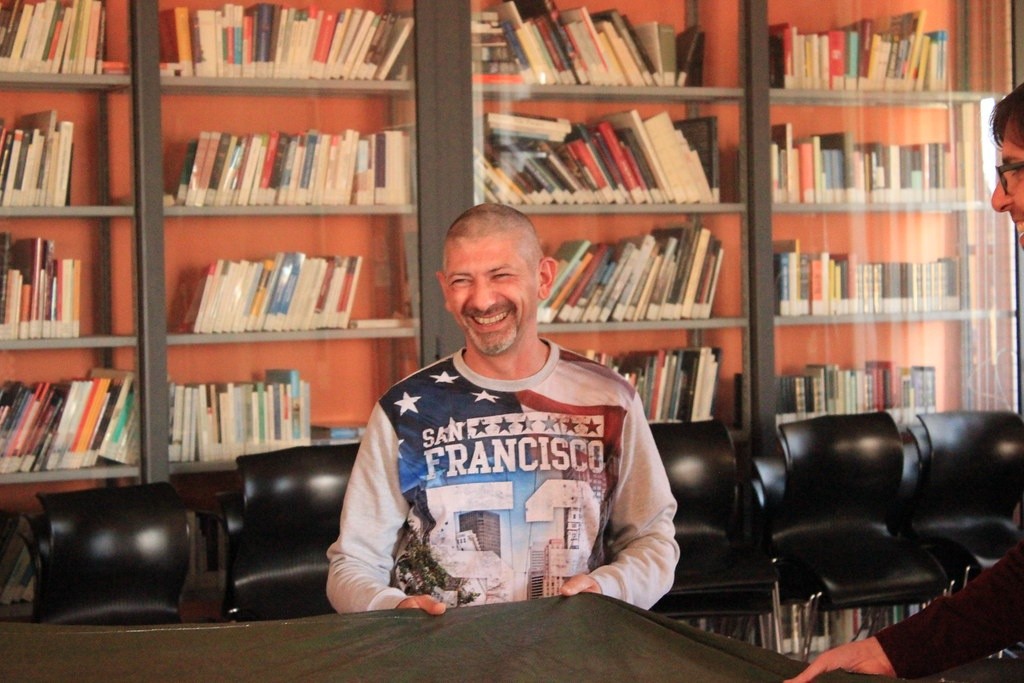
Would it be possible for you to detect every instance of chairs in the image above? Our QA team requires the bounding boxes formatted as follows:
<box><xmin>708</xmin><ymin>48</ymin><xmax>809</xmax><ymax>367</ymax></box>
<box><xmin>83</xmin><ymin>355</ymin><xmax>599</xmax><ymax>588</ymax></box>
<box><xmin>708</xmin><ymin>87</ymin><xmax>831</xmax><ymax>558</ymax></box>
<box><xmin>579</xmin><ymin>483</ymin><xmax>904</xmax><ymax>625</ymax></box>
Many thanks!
<box><xmin>19</xmin><ymin>410</ymin><xmax>1024</xmax><ymax>664</ymax></box>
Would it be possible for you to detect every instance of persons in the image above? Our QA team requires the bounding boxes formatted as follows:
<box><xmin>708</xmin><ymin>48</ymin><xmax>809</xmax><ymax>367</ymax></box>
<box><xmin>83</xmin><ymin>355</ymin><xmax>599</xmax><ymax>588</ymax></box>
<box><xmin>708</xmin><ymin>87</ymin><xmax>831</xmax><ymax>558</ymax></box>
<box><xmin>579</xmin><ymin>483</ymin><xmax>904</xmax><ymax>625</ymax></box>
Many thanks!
<box><xmin>783</xmin><ymin>84</ymin><xmax>1024</xmax><ymax>683</ymax></box>
<box><xmin>325</xmin><ymin>201</ymin><xmax>680</xmax><ymax>615</ymax></box>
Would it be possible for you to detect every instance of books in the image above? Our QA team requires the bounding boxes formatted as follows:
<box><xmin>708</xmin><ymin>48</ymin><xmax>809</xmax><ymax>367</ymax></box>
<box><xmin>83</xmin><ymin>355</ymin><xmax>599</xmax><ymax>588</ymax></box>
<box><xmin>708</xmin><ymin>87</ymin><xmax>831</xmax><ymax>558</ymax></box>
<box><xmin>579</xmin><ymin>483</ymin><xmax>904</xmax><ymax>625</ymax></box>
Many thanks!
<box><xmin>0</xmin><ymin>0</ymin><xmax>957</xmax><ymax>473</ymax></box>
<box><xmin>677</xmin><ymin>581</ymin><xmax>933</xmax><ymax>657</ymax></box>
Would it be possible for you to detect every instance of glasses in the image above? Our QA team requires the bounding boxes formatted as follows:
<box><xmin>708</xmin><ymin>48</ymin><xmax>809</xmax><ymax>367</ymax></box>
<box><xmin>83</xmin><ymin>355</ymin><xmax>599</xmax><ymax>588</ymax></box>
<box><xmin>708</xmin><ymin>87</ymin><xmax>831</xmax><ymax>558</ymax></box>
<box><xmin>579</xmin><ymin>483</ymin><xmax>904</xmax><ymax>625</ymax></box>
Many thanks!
<box><xmin>995</xmin><ymin>162</ymin><xmax>1024</xmax><ymax>196</ymax></box>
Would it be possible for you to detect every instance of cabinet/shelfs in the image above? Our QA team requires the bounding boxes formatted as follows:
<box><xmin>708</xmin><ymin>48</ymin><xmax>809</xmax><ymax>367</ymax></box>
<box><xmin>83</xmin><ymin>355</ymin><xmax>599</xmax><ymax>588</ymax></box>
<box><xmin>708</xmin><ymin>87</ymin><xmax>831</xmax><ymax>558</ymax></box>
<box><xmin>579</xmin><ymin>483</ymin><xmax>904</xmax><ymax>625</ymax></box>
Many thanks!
<box><xmin>0</xmin><ymin>1</ymin><xmax>1024</xmax><ymax>623</ymax></box>
